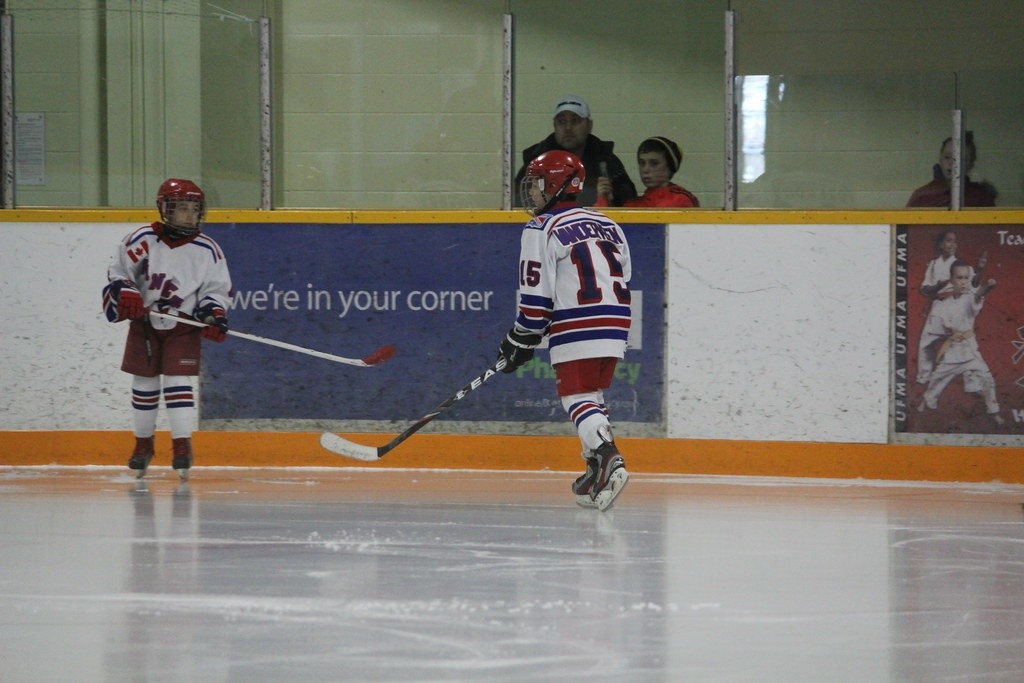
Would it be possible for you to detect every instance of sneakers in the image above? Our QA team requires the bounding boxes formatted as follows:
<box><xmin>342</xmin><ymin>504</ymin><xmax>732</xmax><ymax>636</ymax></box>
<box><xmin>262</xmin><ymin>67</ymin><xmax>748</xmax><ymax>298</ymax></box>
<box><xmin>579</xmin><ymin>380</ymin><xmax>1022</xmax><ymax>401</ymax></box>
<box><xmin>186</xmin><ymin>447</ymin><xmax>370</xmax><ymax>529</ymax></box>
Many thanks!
<box><xmin>128</xmin><ymin>436</ymin><xmax>155</xmax><ymax>479</ymax></box>
<box><xmin>172</xmin><ymin>437</ymin><xmax>192</xmax><ymax>478</ymax></box>
<box><xmin>571</xmin><ymin>457</ymin><xmax>601</xmax><ymax>506</ymax></box>
<box><xmin>589</xmin><ymin>424</ymin><xmax>629</xmax><ymax>512</ymax></box>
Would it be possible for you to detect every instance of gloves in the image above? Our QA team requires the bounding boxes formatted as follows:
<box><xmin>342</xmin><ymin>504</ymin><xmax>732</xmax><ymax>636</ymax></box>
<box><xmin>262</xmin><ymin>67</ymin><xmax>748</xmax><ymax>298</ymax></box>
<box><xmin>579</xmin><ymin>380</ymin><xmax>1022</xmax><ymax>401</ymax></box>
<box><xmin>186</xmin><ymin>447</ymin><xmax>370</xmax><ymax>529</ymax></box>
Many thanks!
<box><xmin>192</xmin><ymin>302</ymin><xmax>228</xmax><ymax>344</ymax></box>
<box><xmin>102</xmin><ymin>281</ymin><xmax>143</xmax><ymax>322</ymax></box>
<box><xmin>497</xmin><ymin>327</ymin><xmax>544</xmax><ymax>374</ymax></box>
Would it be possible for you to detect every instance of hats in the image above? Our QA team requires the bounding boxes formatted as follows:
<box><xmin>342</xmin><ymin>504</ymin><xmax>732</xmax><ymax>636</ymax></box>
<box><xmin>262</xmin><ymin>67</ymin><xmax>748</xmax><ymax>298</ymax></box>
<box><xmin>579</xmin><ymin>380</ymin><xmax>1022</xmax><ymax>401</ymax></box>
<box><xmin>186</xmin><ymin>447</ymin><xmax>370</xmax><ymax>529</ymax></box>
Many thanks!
<box><xmin>551</xmin><ymin>96</ymin><xmax>591</xmax><ymax>119</ymax></box>
<box><xmin>648</xmin><ymin>136</ymin><xmax>683</xmax><ymax>171</ymax></box>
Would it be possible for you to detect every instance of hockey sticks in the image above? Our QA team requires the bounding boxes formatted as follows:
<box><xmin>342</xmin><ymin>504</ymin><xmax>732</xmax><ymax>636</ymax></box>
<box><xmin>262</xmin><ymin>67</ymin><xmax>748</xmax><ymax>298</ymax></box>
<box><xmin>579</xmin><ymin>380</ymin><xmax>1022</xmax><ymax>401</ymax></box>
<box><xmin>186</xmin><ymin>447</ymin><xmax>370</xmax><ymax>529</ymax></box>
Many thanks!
<box><xmin>600</xmin><ymin>161</ymin><xmax>616</xmax><ymax>207</ymax></box>
<box><xmin>319</xmin><ymin>326</ymin><xmax>551</xmax><ymax>463</ymax></box>
<box><xmin>145</xmin><ymin>307</ymin><xmax>396</xmax><ymax>368</ymax></box>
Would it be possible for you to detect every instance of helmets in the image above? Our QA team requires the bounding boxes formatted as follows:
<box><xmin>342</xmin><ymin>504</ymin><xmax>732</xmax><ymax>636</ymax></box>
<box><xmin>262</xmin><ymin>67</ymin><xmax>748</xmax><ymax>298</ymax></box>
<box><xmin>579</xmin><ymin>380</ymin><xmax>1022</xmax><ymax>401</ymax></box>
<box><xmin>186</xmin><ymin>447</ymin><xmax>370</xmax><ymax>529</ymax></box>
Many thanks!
<box><xmin>521</xmin><ymin>150</ymin><xmax>586</xmax><ymax>216</ymax></box>
<box><xmin>156</xmin><ymin>178</ymin><xmax>205</xmax><ymax>236</ymax></box>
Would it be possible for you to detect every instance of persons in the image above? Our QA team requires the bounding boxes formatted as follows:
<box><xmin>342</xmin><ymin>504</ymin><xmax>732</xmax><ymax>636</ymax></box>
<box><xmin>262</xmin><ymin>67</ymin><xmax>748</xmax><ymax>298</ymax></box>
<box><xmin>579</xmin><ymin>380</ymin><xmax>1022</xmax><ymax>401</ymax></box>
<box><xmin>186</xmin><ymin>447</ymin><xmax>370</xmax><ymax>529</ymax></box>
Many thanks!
<box><xmin>907</xmin><ymin>130</ymin><xmax>999</xmax><ymax>207</ymax></box>
<box><xmin>915</xmin><ymin>231</ymin><xmax>1006</xmax><ymax>425</ymax></box>
<box><xmin>103</xmin><ymin>178</ymin><xmax>233</xmax><ymax>480</ymax></box>
<box><xmin>128</xmin><ymin>487</ymin><xmax>194</xmax><ymax>596</ymax></box>
<box><xmin>497</xmin><ymin>152</ymin><xmax>632</xmax><ymax>512</ymax></box>
<box><xmin>515</xmin><ymin>96</ymin><xmax>637</xmax><ymax>208</ymax></box>
<box><xmin>592</xmin><ymin>137</ymin><xmax>700</xmax><ymax>207</ymax></box>
